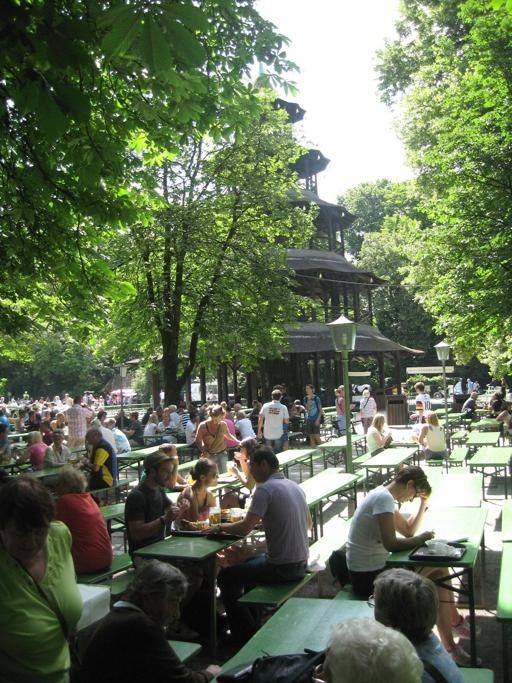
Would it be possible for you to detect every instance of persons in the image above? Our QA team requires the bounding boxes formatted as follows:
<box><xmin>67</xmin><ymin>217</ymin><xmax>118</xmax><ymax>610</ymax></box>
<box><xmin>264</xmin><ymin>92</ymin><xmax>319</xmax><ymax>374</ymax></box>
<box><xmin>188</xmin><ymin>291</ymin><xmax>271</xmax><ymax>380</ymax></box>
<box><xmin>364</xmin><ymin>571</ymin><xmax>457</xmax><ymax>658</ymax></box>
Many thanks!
<box><xmin>323</xmin><ymin>617</ymin><xmax>424</xmax><ymax>682</ymax></box>
<box><xmin>373</xmin><ymin>569</ymin><xmax>464</xmax><ymax>682</ymax></box>
<box><xmin>80</xmin><ymin>558</ymin><xmax>223</xmax><ymax>682</ymax></box>
<box><xmin>1</xmin><ymin>382</ymin><xmax>512</xmax><ymax>666</ymax></box>
<box><xmin>1</xmin><ymin>475</ymin><xmax>84</xmax><ymax>682</ymax></box>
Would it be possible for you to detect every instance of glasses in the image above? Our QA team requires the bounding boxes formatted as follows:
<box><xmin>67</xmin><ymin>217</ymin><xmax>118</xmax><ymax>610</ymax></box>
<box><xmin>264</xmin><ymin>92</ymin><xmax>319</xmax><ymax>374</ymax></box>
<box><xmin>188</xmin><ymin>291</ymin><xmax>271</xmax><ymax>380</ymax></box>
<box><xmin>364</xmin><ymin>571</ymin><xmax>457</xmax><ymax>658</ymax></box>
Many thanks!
<box><xmin>311</xmin><ymin>663</ymin><xmax>326</xmax><ymax>683</ymax></box>
<box><xmin>368</xmin><ymin>595</ymin><xmax>385</xmax><ymax>613</ymax></box>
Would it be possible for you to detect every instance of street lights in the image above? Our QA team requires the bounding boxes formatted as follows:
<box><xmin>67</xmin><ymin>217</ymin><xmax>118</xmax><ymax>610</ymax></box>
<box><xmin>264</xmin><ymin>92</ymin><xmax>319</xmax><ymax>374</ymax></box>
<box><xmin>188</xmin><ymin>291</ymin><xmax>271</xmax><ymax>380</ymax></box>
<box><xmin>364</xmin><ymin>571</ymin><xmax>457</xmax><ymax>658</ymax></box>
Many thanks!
<box><xmin>112</xmin><ymin>358</ymin><xmax>131</xmax><ymax>429</ymax></box>
<box><xmin>326</xmin><ymin>312</ymin><xmax>361</xmax><ymax>473</ymax></box>
<box><xmin>430</xmin><ymin>340</ymin><xmax>455</xmax><ymax>469</ymax></box>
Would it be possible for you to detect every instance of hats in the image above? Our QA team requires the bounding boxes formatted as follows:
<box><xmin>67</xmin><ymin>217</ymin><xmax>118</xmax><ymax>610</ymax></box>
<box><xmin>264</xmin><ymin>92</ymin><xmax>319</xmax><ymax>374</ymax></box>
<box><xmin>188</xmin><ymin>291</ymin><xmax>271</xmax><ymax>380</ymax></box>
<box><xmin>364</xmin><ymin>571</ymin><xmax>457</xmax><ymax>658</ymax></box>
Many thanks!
<box><xmin>143</xmin><ymin>449</ymin><xmax>176</xmax><ymax>473</ymax></box>
<box><xmin>294</xmin><ymin>399</ymin><xmax>301</xmax><ymax>408</ymax></box>
<box><xmin>362</xmin><ymin>390</ymin><xmax>371</xmax><ymax>398</ymax></box>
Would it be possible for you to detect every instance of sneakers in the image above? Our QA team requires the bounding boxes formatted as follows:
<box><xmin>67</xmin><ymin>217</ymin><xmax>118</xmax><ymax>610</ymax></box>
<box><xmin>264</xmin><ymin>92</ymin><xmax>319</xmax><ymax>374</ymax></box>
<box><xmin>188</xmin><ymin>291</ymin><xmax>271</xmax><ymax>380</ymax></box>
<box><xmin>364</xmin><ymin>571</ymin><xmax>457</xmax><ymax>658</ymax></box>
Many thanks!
<box><xmin>448</xmin><ymin>647</ymin><xmax>489</xmax><ymax>667</ymax></box>
<box><xmin>453</xmin><ymin>620</ymin><xmax>484</xmax><ymax>640</ymax></box>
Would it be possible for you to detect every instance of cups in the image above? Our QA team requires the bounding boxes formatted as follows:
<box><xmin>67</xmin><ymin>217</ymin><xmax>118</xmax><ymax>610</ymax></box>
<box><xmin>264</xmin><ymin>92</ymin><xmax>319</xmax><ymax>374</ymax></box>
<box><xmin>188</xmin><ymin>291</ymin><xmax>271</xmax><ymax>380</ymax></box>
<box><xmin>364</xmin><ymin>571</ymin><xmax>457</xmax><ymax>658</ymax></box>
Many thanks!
<box><xmin>209</xmin><ymin>506</ymin><xmax>221</xmax><ymax>527</ymax></box>
<box><xmin>230</xmin><ymin>508</ymin><xmax>242</xmax><ymax>524</ymax></box>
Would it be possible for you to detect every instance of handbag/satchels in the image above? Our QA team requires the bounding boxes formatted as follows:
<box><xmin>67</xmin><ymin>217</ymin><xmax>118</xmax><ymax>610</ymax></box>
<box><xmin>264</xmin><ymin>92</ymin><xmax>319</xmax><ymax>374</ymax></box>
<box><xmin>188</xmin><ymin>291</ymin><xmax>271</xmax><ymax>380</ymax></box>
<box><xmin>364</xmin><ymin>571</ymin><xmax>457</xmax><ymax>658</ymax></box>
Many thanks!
<box><xmin>330</xmin><ymin>545</ymin><xmax>351</xmax><ymax>587</ymax></box>
<box><xmin>68</xmin><ymin>634</ymin><xmax>83</xmax><ymax>671</ymax></box>
<box><xmin>250</xmin><ymin>649</ymin><xmax>328</xmax><ymax>683</ymax></box>
